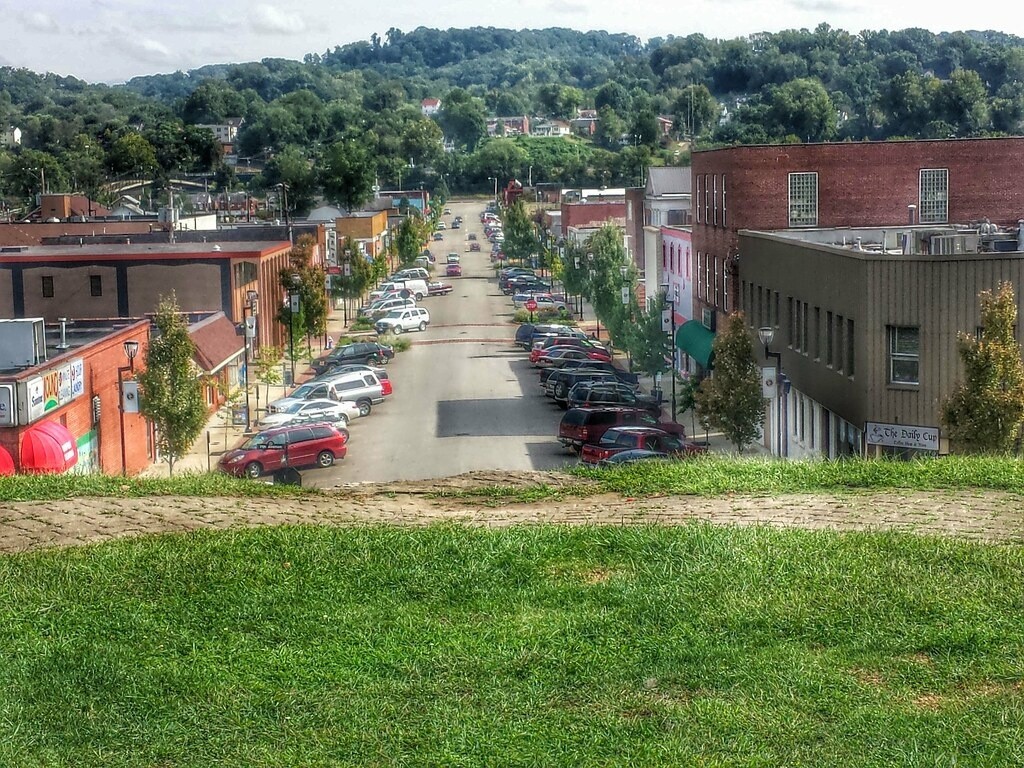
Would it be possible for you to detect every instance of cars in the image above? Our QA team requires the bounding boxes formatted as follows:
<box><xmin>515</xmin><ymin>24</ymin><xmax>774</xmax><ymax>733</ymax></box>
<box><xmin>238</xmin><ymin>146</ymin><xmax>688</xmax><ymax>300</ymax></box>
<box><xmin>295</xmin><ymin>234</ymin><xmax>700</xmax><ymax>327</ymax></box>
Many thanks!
<box><xmin>599</xmin><ymin>426</ymin><xmax>668</xmax><ymax>444</ymax></box>
<box><xmin>361</xmin><ymin>292</ymin><xmax>416</xmax><ymax>322</ymax></box>
<box><xmin>395</xmin><ymin>272</ymin><xmax>429</xmax><ymax>283</ymax></box>
<box><xmin>220</xmin><ymin>424</ymin><xmax>347</xmax><ymax>479</ymax></box>
<box><xmin>303</xmin><ymin>364</ymin><xmax>389</xmax><ymax>380</ymax></box>
<box><xmin>452</xmin><ymin>221</ymin><xmax>459</xmax><ymax>229</ymax></box>
<box><xmin>444</xmin><ymin>208</ymin><xmax>451</xmax><ymax>215</ymax></box>
<box><xmin>515</xmin><ymin>324</ymin><xmax>639</xmax><ymax>411</ymax></box>
<box><xmin>481</xmin><ymin>212</ymin><xmax>506</xmax><ymax>262</ymax></box>
<box><xmin>469</xmin><ymin>242</ymin><xmax>481</xmax><ymax>252</ymax></box>
<box><xmin>257</xmin><ymin>398</ymin><xmax>360</xmax><ymax>424</ymax></box>
<box><xmin>390</xmin><ymin>267</ymin><xmax>430</xmax><ymax>277</ymax></box>
<box><xmin>447</xmin><ymin>252</ymin><xmax>460</xmax><ymax>264</ymax></box>
<box><xmin>568</xmin><ymin>380</ymin><xmax>661</xmax><ymax>418</ymax></box>
<box><xmin>596</xmin><ymin>449</ymin><xmax>677</xmax><ymax>470</ymax></box>
<box><xmin>413</xmin><ymin>250</ymin><xmax>435</xmax><ymax>267</ymax></box>
<box><xmin>378</xmin><ymin>345</ymin><xmax>394</xmax><ymax>365</ymax></box>
<box><xmin>284</xmin><ymin>410</ymin><xmax>349</xmax><ymax>444</ymax></box>
<box><xmin>310</xmin><ymin>342</ymin><xmax>383</xmax><ymax>374</ymax></box>
<box><xmin>426</xmin><ymin>281</ymin><xmax>454</xmax><ymax>296</ymax></box>
<box><xmin>455</xmin><ymin>217</ymin><xmax>462</xmax><ymax>223</ymax></box>
<box><xmin>438</xmin><ymin>222</ymin><xmax>446</xmax><ymax>230</ymax></box>
<box><xmin>446</xmin><ymin>265</ymin><xmax>461</xmax><ymax>276</ymax></box>
<box><xmin>468</xmin><ymin>233</ymin><xmax>477</xmax><ymax>240</ymax></box>
<box><xmin>434</xmin><ymin>233</ymin><xmax>443</xmax><ymax>241</ymax></box>
<box><xmin>498</xmin><ymin>267</ymin><xmax>566</xmax><ymax>311</ymax></box>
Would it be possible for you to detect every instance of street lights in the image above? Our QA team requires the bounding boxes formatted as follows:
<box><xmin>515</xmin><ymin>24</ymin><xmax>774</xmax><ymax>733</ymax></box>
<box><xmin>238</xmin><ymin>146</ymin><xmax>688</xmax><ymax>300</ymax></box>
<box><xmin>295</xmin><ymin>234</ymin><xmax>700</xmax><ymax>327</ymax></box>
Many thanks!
<box><xmin>587</xmin><ymin>252</ymin><xmax>600</xmax><ymax>339</ymax></box>
<box><xmin>545</xmin><ymin>229</ymin><xmax>553</xmax><ymax>288</ymax></box>
<box><xmin>488</xmin><ymin>177</ymin><xmax>497</xmax><ymax>209</ymax></box>
<box><xmin>573</xmin><ymin>242</ymin><xmax>579</xmax><ymax>314</ymax></box>
<box><xmin>659</xmin><ymin>283</ymin><xmax>677</xmax><ymax>423</ymax></box>
<box><xmin>357</xmin><ymin>241</ymin><xmax>365</xmax><ymax>309</ymax></box>
<box><xmin>321</xmin><ymin>259</ymin><xmax>332</xmax><ymax>350</ymax></box>
<box><xmin>342</xmin><ymin>249</ymin><xmax>350</xmax><ymax>328</ymax></box>
<box><xmin>384</xmin><ymin>219</ymin><xmax>403</xmax><ymax>279</ymax></box>
<box><xmin>559</xmin><ymin>233</ymin><xmax>568</xmax><ymax>302</ymax></box>
<box><xmin>242</xmin><ymin>290</ymin><xmax>258</xmax><ymax>432</ymax></box>
<box><xmin>759</xmin><ymin>327</ymin><xmax>782</xmax><ymax>460</ymax></box>
<box><xmin>620</xmin><ymin>265</ymin><xmax>633</xmax><ymax>372</ymax></box>
<box><xmin>375</xmin><ymin>233</ymin><xmax>381</xmax><ymax>289</ymax></box>
<box><xmin>117</xmin><ymin>338</ymin><xmax>139</xmax><ymax>477</ymax></box>
<box><xmin>441</xmin><ymin>174</ymin><xmax>449</xmax><ymax>179</ymax></box>
<box><xmin>286</xmin><ymin>274</ymin><xmax>301</xmax><ymax>388</ymax></box>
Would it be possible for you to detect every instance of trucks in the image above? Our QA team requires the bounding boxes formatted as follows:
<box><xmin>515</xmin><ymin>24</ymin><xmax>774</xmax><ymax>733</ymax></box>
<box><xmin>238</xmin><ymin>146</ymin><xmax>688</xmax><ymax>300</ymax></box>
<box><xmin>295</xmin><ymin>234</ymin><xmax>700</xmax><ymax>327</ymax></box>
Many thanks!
<box><xmin>370</xmin><ymin>280</ymin><xmax>428</xmax><ymax>301</ymax></box>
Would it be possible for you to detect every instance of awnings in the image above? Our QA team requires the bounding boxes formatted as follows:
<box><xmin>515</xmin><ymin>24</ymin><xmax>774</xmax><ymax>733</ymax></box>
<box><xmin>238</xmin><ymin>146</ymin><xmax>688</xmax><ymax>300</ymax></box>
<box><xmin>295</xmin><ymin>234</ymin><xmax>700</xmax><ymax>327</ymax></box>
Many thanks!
<box><xmin>189</xmin><ymin>316</ymin><xmax>250</xmax><ymax>376</ymax></box>
<box><xmin>674</xmin><ymin>320</ymin><xmax>717</xmax><ymax>370</ymax></box>
<box><xmin>21</xmin><ymin>420</ymin><xmax>79</xmax><ymax>474</ymax></box>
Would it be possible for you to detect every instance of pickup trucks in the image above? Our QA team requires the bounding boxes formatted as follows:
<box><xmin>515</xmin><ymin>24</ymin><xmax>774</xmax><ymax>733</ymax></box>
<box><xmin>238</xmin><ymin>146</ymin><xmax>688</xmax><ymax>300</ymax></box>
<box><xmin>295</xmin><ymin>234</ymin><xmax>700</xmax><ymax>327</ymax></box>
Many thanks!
<box><xmin>582</xmin><ymin>430</ymin><xmax>707</xmax><ymax>463</ymax></box>
<box><xmin>265</xmin><ymin>370</ymin><xmax>385</xmax><ymax>417</ymax></box>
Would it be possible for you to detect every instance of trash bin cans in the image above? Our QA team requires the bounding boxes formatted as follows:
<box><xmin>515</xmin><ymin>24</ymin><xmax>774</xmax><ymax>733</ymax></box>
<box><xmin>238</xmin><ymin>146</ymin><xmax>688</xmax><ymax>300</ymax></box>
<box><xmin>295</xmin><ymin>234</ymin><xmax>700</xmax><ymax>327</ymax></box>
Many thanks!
<box><xmin>273</xmin><ymin>466</ymin><xmax>301</xmax><ymax>487</ymax></box>
<box><xmin>232</xmin><ymin>405</ymin><xmax>248</xmax><ymax>425</ymax></box>
<box><xmin>282</xmin><ymin>370</ymin><xmax>293</xmax><ymax>384</ymax></box>
<box><xmin>651</xmin><ymin>386</ymin><xmax>663</xmax><ymax>405</ymax></box>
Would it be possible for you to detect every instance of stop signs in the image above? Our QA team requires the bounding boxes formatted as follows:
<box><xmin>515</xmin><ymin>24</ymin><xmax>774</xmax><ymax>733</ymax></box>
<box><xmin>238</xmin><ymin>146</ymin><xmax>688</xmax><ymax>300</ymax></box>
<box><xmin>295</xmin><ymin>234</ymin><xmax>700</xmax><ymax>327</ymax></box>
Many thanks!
<box><xmin>524</xmin><ymin>300</ymin><xmax>537</xmax><ymax>311</ymax></box>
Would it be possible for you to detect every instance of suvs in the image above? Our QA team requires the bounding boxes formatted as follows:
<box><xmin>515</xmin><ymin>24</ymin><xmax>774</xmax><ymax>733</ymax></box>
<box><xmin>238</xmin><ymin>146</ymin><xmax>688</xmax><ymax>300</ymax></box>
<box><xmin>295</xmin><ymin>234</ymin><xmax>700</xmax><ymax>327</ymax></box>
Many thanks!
<box><xmin>558</xmin><ymin>403</ymin><xmax>684</xmax><ymax>453</ymax></box>
<box><xmin>374</xmin><ymin>308</ymin><xmax>430</xmax><ymax>335</ymax></box>
